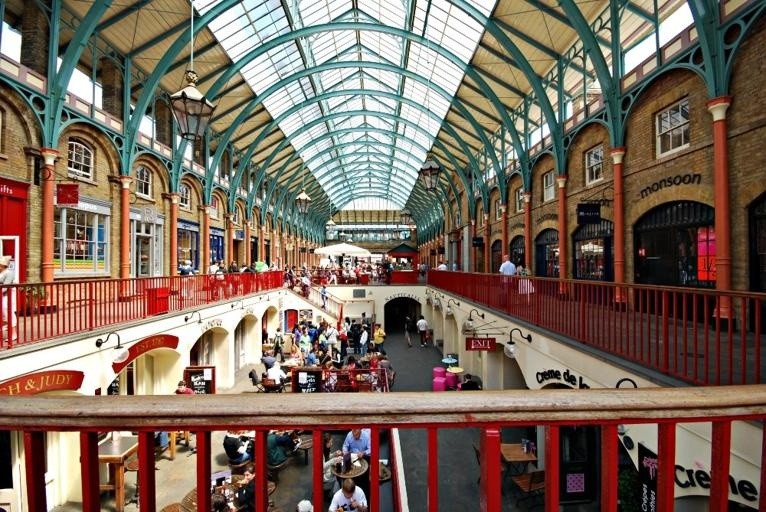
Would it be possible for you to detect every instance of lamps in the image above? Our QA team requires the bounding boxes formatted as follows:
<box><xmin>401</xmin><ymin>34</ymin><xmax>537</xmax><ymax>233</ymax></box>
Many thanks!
<box><xmin>400</xmin><ymin>0</ymin><xmax>441</xmax><ymax>225</ymax></box>
<box><xmin>293</xmin><ymin>36</ymin><xmax>336</xmax><ymax>241</ymax></box>
<box><xmin>98</xmin><ymin>287</ymin><xmax>292</xmax><ymax>366</ymax></box>
<box><xmin>422</xmin><ymin>286</ymin><xmax>531</xmax><ymax>359</ymax></box>
<box><xmin>169</xmin><ymin>1</ymin><xmax>217</xmax><ymax>142</ymax></box>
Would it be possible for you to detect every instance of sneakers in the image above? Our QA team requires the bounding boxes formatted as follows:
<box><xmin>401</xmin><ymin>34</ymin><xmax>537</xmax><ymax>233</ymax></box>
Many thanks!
<box><xmin>408</xmin><ymin>342</ymin><xmax>428</xmax><ymax>348</ymax></box>
<box><xmin>292</xmin><ymin>438</ymin><xmax>302</xmax><ymax>452</ymax></box>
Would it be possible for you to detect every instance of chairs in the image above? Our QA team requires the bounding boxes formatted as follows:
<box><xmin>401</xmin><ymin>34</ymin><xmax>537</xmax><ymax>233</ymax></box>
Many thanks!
<box><xmin>470</xmin><ymin>436</ymin><xmax>546</xmax><ymax>511</ymax></box>
<box><xmin>248</xmin><ymin>369</ymin><xmax>285</xmax><ymax>392</ymax></box>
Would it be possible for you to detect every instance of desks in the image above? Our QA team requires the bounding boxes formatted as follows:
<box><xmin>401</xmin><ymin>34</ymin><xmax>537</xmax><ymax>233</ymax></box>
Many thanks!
<box><xmin>331</xmin><ymin>454</ymin><xmax>368</xmax><ymax>494</ymax></box>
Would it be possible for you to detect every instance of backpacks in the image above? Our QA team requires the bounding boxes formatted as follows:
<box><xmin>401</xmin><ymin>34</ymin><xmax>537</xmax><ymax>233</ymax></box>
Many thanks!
<box><xmin>216</xmin><ymin>269</ymin><xmax>224</xmax><ymax>280</ymax></box>
<box><xmin>352</xmin><ymin>324</ymin><xmax>360</xmax><ymax>337</ymax></box>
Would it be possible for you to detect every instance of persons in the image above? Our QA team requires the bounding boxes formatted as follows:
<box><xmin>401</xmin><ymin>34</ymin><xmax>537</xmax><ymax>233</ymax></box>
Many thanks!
<box><xmin>499</xmin><ymin>255</ymin><xmax>517</xmax><ymax>304</ymax></box>
<box><xmin>172</xmin><ymin>380</ymin><xmax>194</xmax><ymax>395</ymax></box>
<box><xmin>153</xmin><ymin>431</ymin><xmax>170</xmax><ymax>460</ymax></box>
<box><xmin>461</xmin><ymin>374</ymin><xmax>481</xmax><ymax>391</ymax></box>
<box><xmin>320</xmin><ymin>283</ymin><xmax>330</xmax><ymax>310</ymax></box>
<box><xmin>174</xmin><ymin>255</ymin><xmax>427</xmax><ymax>302</ymax></box>
<box><xmin>209</xmin><ymin>308</ymin><xmax>398</xmax><ymax>511</ymax></box>
<box><xmin>431</xmin><ymin>261</ymin><xmax>447</xmax><ymax>270</ymax></box>
<box><xmin>403</xmin><ymin>316</ymin><xmax>413</xmax><ymax>348</ymax></box>
<box><xmin>0</xmin><ymin>254</ymin><xmax>20</xmax><ymax>343</ymax></box>
<box><xmin>416</xmin><ymin>314</ymin><xmax>430</xmax><ymax>348</ymax></box>
<box><xmin>515</xmin><ymin>260</ymin><xmax>535</xmax><ymax>305</ymax></box>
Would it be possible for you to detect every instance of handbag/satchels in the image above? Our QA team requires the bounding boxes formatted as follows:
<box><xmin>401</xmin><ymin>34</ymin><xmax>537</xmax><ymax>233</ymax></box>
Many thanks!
<box><xmin>154</xmin><ymin>437</ymin><xmax>160</xmax><ymax>447</ymax></box>
<box><xmin>339</xmin><ymin>331</ymin><xmax>352</xmax><ymax>341</ymax></box>
<box><xmin>426</xmin><ymin>330</ymin><xmax>431</xmax><ymax>339</ymax></box>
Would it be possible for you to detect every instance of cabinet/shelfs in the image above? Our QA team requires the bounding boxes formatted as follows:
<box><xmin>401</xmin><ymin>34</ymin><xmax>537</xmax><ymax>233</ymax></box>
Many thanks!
<box><xmin>97</xmin><ymin>430</ymin><xmax>194</xmax><ymax>511</ymax></box>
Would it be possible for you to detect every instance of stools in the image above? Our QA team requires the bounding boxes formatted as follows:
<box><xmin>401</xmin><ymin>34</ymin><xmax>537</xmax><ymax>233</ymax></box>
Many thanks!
<box><xmin>432</xmin><ymin>366</ymin><xmax>463</xmax><ymax>390</ymax></box>
<box><xmin>228</xmin><ymin>438</ymin><xmax>313</xmax><ymax>483</ymax></box>
